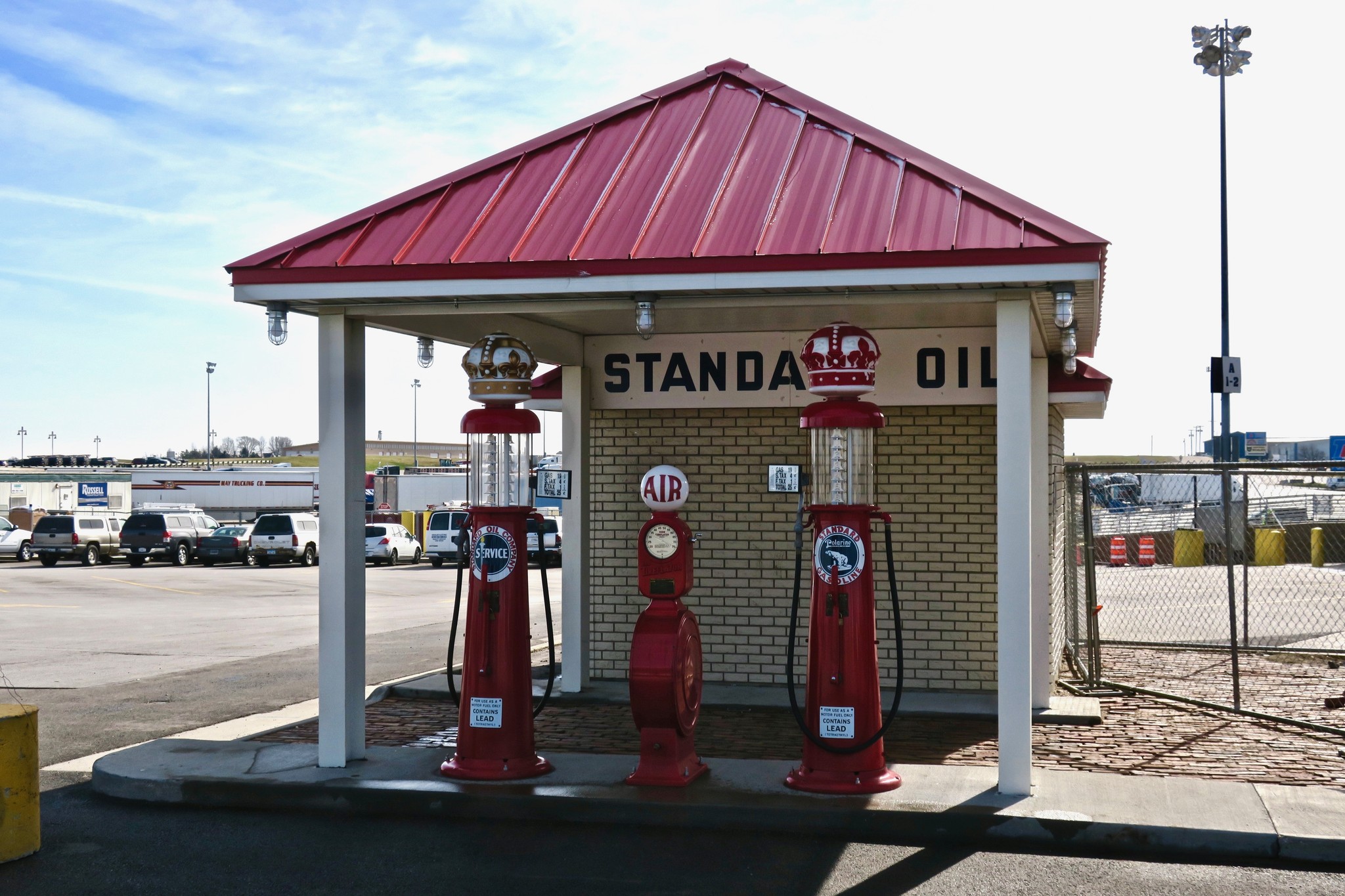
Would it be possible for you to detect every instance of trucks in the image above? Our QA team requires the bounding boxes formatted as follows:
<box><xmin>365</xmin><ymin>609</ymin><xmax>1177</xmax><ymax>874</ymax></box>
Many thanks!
<box><xmin>89</xmin><ymin>456</ymin><xmax>118</xmax><ymax>466</ymax></box>
<box><xmin>452</xmin><ymin>460</ymin><xmax>471</xmax><ymax>472</ymax></box>
<box><xmin>76</xmin><ymin>457</ymin><xmax>85</xmax><ymax>466</ymax></box>
<box><xmin>373</xmin><ymin>466</ymin><xmax>400</xmax><ymax>475</ymax></box>
<box><xmin>6</xmin><ymin>457</ymin><xmax>43</xmax><ymax>466</ymax></box>
<box><xmin>533</xmin><ymin>454</ymin><xmax>563</xmax><ymax>473</ymax></box>
<box><xmin>1139</xmin><ymin>472</ymin><xmax>1245</xmax><ymax>505</ymax></box>
<box><xmin>47</xmin><ymin>457</ymin><xmax>58</xmax><ymax>467</ymax></box>
<box><xmin>61</xmin><ymin>456</ymin><xmax>72</xmax><ymax>466</ymax></box>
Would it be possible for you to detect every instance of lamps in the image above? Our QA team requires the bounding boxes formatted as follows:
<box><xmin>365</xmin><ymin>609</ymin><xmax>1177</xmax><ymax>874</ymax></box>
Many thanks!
<box><xmin>417</xmin><ymin>336</ymin><xmax>434</xmax><ymax>368</ymax></box>
<box><xmin>266</xmin><ymin>301</ymin><xmax>289</xmax><ymax>345</ymax></box>
<box><xmin>630</xmin><ymin>291</ymin><xmax>660</xmax><ymax>340</ymax></box>
<box><xmin>1051</xmin><ymin>281</ymin><xmax>1077</xmax><ymax>374</ymax></box>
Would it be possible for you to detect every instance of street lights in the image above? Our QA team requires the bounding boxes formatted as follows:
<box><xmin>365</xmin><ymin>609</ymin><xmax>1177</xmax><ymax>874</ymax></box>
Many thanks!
<box><xmin>205</xmin><ymin>361</ymin><xmax>215</xmax><ymax>470</ymax></box>
<box><xmin>209</xmin><ymin>429</ymin><xmax>217</xmax><ymax>462</ymax></box>
<box><xmin>47</xmin><ymin>431</ymin><xmax>56</xmax><ymax>455</ymax></box>
<box><xmin>1188</xmin><ymin>17</ymin><xmax>1251</xmax><ymax>711</ymax></box>
<box><xmin>17</xmin><ymin>427</ymin><xmax>27</xmax><ymax>468</ymax></box>
<box><xmin>93</xmin><ymin>436</ymin><xmax>101</xmax><ymax>458</ymax></box>
<box><xmin>407</xmin><ymin>379</ymin><xmax>422</xmax><ymax>470</ymax></box>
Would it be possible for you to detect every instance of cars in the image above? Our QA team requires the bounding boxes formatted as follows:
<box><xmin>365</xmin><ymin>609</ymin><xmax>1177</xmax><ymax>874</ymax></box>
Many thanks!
<box><xmin>1077</xmin><ymin>472</ymin><xmax>1139</xmax><ymax>511</ymax></box>
<box><xmin>0</xmin><ymin>516</ymin><xmax>33</xmax><ymax>562</ymax></box>
<box><xmin>195</xmin><ymin>526</ymin><xmax>253</xmax><ymax>567</ymax></box>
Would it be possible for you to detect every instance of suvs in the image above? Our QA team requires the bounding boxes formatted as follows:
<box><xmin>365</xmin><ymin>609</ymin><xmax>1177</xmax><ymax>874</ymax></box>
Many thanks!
<box><xmin>522</xmin><ymin>517</ymin><xmax>563</xmax><ymax>566</ymax></box>
<box><xmin>132</xmin><ymin>457</ymin><xmax>188</xmax><ymax>465</ymax></box>
<box><xmin>118</xmin><ymin>502</ymin><xmax>220</xmax><ymax>564</ymax></box>
<box><xmin>363</xmin><ymin>523</ymin><xmax>421</xmax><ymax>565</ymax></box>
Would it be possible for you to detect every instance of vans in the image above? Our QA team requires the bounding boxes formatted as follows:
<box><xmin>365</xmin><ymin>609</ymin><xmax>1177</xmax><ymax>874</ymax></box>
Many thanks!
<box><xmin>244</xmin><ymin>513</ymin><xmax>322</xmax><ymax>567</ymax></box>
<box><xmin>422</xmin><ymin>510</ymin><xmax>473</xmax><ymax>570</ymax></box>
<box><xmin>1326</xmin><ymin>477</ymin><xmax>1345</xmax><ymax>489</ymax></box>
<box><xmin>30</xmin><ymin>515</ymin><xmax>131</xmax><ymax>564</ymax></box>
